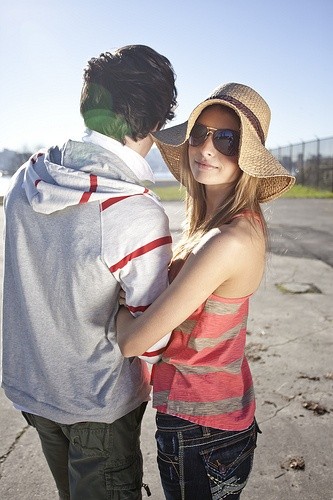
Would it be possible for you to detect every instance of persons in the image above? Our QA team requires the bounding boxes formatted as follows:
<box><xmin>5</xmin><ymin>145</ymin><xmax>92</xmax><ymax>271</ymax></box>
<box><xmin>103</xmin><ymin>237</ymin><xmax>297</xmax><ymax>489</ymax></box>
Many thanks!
<box><xmin>2</xmin><ymin>43</ymin><xmax>172</xmax><ymax>500</ymax></box>
<box><xmin>113</xmin><ymin>82</ymin><xmax>296</xmax><ymax>498</ymax></box>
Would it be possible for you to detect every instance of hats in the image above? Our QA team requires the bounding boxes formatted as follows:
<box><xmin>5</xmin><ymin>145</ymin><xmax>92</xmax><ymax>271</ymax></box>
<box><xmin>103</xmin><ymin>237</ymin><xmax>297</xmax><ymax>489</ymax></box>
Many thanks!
<box><xmin>149</xmin><ymin>82</ymin><xmax>296</xmax><ymax>204</ymax></box>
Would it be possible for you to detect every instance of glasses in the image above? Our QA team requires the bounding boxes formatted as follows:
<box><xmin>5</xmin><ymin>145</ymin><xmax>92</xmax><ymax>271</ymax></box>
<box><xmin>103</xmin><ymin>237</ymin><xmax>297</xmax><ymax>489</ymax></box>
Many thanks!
<box><xmin>188</xmin><ymin>122</ymin><xmax>240</xmax><ymax>156</ymax></box>
